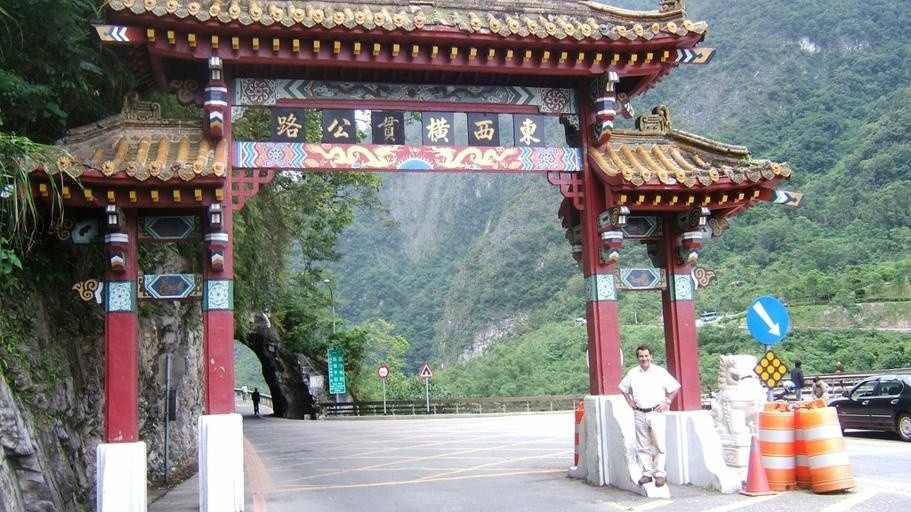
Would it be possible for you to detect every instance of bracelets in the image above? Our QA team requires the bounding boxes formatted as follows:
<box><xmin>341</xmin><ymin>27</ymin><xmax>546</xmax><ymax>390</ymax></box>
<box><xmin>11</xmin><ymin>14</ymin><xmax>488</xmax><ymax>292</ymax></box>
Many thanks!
<box><xmin>665</xmin><ymin>399</ymin><xmax>671</xmax><ymax>404</ymax></box>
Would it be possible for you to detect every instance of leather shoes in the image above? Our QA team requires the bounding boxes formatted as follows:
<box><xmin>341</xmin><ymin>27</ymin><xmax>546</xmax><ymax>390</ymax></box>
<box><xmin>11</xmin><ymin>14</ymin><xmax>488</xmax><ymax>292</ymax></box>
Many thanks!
<box><xmin>638</xmin><ymin>475</ymin><xmax>652</xmax><ymax>485</ymax></box>
<box><xmin>654</xmin><ymin>477</ymin><xmax>667</xmax><ymax>487</ymax></box>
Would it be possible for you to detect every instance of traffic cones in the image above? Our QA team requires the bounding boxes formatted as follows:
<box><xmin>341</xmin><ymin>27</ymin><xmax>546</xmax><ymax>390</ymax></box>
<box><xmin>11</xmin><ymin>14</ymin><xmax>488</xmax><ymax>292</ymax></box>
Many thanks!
<box><xmin>739</xmin><ymin>436</ymin><xmax>777</xmax><ymax>498</ymax></box>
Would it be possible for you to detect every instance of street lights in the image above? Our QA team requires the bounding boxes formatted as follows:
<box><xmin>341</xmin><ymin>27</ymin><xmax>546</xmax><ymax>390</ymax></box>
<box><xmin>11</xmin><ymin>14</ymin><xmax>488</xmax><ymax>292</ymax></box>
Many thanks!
<box><xmin>324</xmin><ymin>279</ymin><xmax>338</xmax><ymax>417</ymax></box>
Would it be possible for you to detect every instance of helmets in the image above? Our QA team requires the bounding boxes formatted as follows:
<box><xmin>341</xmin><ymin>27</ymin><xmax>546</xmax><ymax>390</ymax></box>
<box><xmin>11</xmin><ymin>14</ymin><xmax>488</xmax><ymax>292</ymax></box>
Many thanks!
<box><xmin>783</xmin><ymin>381</ymin><xmax>796</xmax><ymax>394</ymax></box>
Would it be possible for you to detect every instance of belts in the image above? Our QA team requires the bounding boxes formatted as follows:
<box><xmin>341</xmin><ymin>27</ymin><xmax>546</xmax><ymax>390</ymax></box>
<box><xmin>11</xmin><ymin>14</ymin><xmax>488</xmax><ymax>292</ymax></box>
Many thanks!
<box><xmin>635</xmin><ymin>407</ymin><xmax>655</xmax><ymax>413</ymax></box>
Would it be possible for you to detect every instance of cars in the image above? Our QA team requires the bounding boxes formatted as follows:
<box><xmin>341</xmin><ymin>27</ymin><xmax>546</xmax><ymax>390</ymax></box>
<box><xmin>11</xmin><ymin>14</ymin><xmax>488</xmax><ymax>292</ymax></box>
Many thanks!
<box><xmin>823</xmin><ymin>375</ymin><xmax>911</xmax><ymax>442</ymax></box>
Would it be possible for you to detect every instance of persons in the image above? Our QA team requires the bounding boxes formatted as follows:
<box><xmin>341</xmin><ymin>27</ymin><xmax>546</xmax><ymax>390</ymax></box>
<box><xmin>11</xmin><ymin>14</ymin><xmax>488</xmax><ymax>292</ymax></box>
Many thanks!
<box><xmin>836</xmin><ymin>362</ymin><xmax>844</xmax><ymax>388</ymax></box>
<box><xmin>618</xmin><ymin>344</ymin><xmax>681</xmax><ymax>487</ymax></box>
<box><xmin>252</xmin><ymin>388</ymin><xmax>260</xmax><ymax>416</ymax></box>
<box><xmin>791</xmin><ymin>360</ymin><xmax>805</xmax><ymax>401</ymax></box>
<box><xmin>241</xmin><ymin>383</ymin><xmax>247</xmax><ymax>401</ymax></box>
<box><xmin>811</xmin><ymin>377</ymin><xmax>831</xmax><ymax>400</ymax></box>
